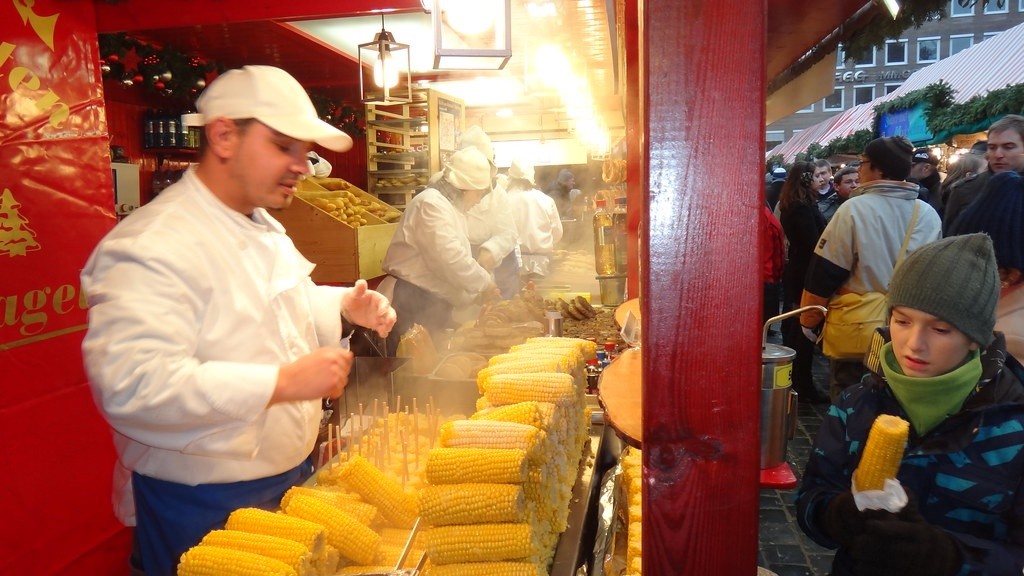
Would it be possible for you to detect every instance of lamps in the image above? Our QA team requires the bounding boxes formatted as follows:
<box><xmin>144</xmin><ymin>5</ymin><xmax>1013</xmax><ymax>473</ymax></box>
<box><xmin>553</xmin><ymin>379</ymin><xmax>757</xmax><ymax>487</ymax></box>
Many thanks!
<box><xmin>358</xmin><ymin>15</ymin><xmax>412</xmax><ymax>106</ymax></box>
<box><xmin>432</xmin><ymin>1</ymin><xmax>512</xmax><ymax>71</ymax></box>
<box><xmin>883</xmin><ymin>0</ymin><xmax>904</xmax><ymax>21</ymax></box>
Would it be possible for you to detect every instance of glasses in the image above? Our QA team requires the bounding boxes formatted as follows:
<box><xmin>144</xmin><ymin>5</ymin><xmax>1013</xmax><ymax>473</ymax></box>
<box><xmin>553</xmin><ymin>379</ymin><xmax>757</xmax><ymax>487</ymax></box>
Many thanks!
<box><xmin>857</xmin><ymin>159</ymin><xmax>872</xmax><ymax>166</ymax></box>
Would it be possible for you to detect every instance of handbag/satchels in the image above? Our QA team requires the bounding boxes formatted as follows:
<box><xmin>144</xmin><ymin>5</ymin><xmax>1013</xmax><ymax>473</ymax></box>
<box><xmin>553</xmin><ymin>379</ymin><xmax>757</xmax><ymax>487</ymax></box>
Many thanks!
<box><xmin>816</xmin><ymin>293</ymin><xmax>889</xmax><ymax>360</ymax></box>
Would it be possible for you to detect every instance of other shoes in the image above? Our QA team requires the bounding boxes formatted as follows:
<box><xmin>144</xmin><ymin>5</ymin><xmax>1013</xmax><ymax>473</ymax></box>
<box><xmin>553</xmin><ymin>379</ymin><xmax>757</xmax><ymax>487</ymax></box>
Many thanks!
<box><xmin>790</xmin><ymin>372</ymin><xmax>818</xmax><ymax>402</ymax></box>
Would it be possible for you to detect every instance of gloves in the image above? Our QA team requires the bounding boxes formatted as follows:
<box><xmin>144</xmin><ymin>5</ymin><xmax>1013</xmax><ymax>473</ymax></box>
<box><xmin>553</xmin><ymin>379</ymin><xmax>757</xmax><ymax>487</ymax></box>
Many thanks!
<box><xmin>801</xmin><ymin>325</ymin><xmax>819</xmax><ymax>343</ymax></box>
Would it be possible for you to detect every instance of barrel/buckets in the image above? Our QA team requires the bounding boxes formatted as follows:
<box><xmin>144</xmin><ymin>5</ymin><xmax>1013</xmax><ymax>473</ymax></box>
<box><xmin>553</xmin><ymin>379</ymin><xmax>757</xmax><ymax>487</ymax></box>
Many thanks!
<box><xmin>596</xmin><ymin>275</ymin><xmax>628</xmax><ymax>306</ymax></box>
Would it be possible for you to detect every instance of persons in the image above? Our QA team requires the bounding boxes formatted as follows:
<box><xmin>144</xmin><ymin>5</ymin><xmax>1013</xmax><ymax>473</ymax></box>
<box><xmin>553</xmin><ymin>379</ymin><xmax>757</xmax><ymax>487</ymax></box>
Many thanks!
<box><xmin>79</xmin><ymin>64</ymin><xmax>397</xmax><ymax>576</ymax></box>
<box><xmin>765</xmin><ymin>115</ymin><xmax>1024</xmax><ymax>576</ymax></box>
<box><xmin>373</xmin><ymin>122</ymin><xmax>583</xmax><ymax>365</ymax></box>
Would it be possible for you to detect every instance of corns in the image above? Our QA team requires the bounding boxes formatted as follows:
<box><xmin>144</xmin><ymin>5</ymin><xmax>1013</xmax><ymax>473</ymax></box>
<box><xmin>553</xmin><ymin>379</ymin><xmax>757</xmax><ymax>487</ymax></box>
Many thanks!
<box><xmin>176</xmin><ymin>336</ymin><xmax>598</xmax><ymax>576</ymax></box>
<box><xmin>853</xmin><ymin>414</ymin><xmax>910</xmax><ymax>492</ymax></box>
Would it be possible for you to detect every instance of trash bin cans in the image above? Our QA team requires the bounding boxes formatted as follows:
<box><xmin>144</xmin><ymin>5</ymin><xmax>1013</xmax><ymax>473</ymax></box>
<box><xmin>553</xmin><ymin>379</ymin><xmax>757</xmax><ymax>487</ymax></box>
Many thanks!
<box><xmin>759</xmin><ymin>341</ymin><xmax>799</xmax><ymax>488</ymax></box>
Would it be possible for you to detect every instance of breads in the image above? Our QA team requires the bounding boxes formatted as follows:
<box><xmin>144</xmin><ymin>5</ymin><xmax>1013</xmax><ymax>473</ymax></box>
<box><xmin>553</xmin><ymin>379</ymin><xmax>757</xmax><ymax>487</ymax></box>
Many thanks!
<box><xmin>376</xmin><ymin>174</ymin><xmax>430</xmax><ymax>187</ymax></box>
<box><xmin>313</xmin><ymin>181</ymin><xmax>381</xmax><ymax>228</ymax></box>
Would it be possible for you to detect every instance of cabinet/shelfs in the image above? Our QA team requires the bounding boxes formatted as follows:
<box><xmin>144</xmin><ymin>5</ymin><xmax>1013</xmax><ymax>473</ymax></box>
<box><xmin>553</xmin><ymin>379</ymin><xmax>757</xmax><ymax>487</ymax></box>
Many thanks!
<box><xmin>365</xmin><ymin>88</ymin><xmax>465</xmax><ymax>208</ymax></box>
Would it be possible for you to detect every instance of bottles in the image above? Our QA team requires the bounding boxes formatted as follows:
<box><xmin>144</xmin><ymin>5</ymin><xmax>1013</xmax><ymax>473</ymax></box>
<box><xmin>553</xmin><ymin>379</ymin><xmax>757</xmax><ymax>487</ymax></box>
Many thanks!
<box><xmin>168</xmin><ymin>113</ymin><xmax>177</xmax><ymax>147</ymax></box>
<box><xmin>143</xmin><ymin>108</ymin><xmax>156</xmax><ymax>148</ymax></box>
<box><xmin>612</xmin><ymin>197</ymin><xmax>629</xmax><ymax>274</ymax></box>
<box><xmin>593</xmin><ymin>201</ymin><xmax>616</xmax><ymax>275</ymax></box>
<box><xmin>545</xmin><ymin>311</ymin><xmax>563</xmax><ymax>337</ymax></box>
<box><xmin>586</xmin><ymin>358</ymin><xmax>611</xmax><ymax>393</ymax></box>
<box><xmin>596</xmin><ymin>338</ymin><xmax>633</xmax><ymax>361</ymax></box>
<box><xmin>155</xmin><ymin>109</ymin><xmax>167</xmax><ymax>148</ymax></box>
<box><xmin>586</xmin><ymin>337</ymin><xmax>596</xmax><ymax>344</ymax></box>
<box><xmin>177</xmin><ymin>110</ymin><xmax>200</xmax><ymax>150</ymax></box>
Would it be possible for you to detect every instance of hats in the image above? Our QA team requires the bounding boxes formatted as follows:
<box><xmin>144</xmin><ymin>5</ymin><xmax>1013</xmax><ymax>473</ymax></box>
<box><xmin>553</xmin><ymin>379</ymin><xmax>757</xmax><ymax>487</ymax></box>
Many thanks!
<box><xmin>455</xmin><ymin>125</ymin><xmax>499</xmax><ymax>178</ymax></box>
<box><xmin>946</xmin><ymin>170</ymin><xmax>1024</xmax><ymax>273</ymax></box>
<box><xmin>912</xmin><ymin>148</ymin><xmax>940</xmax><ymax>165</ymax></box>
<box><xmin>558</xmin><ymin>168</ymin><xmax>574</xmax><ymax>182</ymax></box>
<box><xmin>444</xmin><ymin>146</ymin><xmax>493</xmax><ymax>190</ymax></box>
<box><xmin>507</xmin><ymin>154</ymin><xmax>536</xmax><ymax>186</ymax></box>
<box><xmin>865</xmin><ymin>135</ymin><xmax>913</xmax><ymax>180</ymax></box>
<box><xmin>182</xmin><ymin>65</ymin><xmax>354</xmax><ymax>152</ymax></box>
<box><xmin>886</xmin><ymin>232</ymin><xmax>1001</xmax><ymax>348</ymax></box>
<box><xmin>496</xmin><ymin>172</ymin><xmax>509</xmax><ymax>191</ymax></box>
<box><xmin>772</xmin><ymin>167</ymin><xmax>787</xmax><ymax>180</ymax></box>
